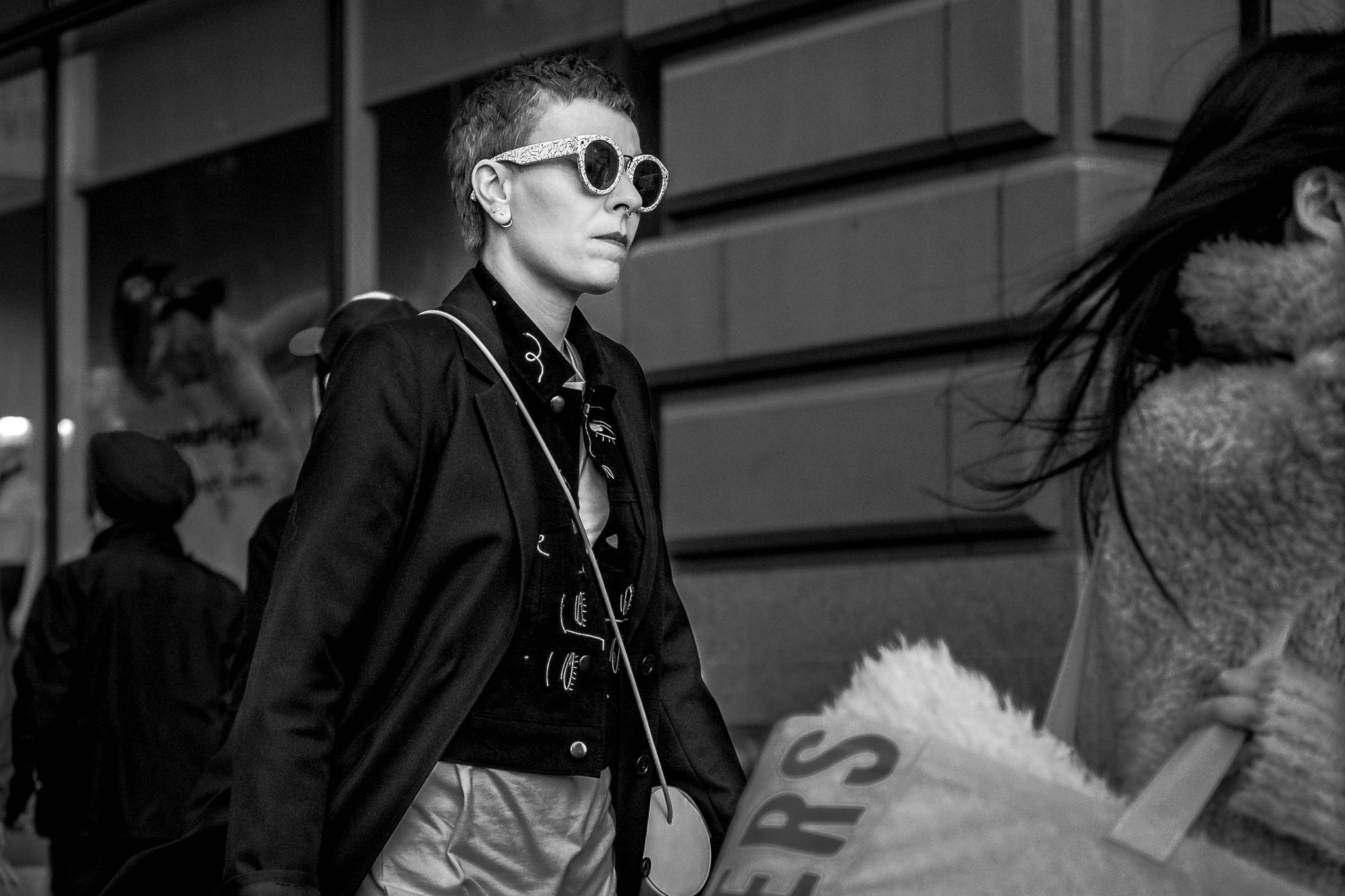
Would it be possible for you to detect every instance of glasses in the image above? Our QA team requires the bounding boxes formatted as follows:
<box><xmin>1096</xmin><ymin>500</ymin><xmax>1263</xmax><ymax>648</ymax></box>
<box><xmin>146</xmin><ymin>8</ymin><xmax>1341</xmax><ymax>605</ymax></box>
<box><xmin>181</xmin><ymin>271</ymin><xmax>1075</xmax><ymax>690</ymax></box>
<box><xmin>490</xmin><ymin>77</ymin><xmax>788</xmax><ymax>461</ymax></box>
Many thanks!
<box><xmin>469</xmin><ymin>134</ymin><xmax>669</xmax><ymax>212</ymax></box>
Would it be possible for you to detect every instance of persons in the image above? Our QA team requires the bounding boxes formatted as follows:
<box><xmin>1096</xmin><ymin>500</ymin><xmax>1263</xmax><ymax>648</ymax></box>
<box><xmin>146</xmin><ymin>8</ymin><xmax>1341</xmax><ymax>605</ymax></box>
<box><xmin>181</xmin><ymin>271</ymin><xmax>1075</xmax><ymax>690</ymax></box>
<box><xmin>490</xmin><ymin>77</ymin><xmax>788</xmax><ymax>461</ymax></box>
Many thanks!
<box><xmin>228</xmin><ymin>49</ymin><xmax>745</xmax><ymax>896</ymax></box>
<box><xmin>0</xmin><ymin>428</ymin><xmax>248</xmax><ymax>896</ymax></box>
<box><xmin>924</xmin><ymin>29</ymin><xmax>1345</xmax><ymax>896</ymax></box>
<box><xmin>244</xmin><ymin>294</ymin><xmax>416</xmax><ymax>595</ymax></box>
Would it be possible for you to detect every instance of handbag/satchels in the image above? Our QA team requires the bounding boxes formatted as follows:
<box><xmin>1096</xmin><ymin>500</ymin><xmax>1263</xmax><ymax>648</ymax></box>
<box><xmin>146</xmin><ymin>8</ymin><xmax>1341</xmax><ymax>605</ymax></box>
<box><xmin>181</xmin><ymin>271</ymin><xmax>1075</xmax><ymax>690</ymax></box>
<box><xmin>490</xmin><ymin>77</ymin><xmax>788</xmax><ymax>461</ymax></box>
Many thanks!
<box><xmin>642</xmin><ymin>786</ymin><xmax>714</xmax><ymax>896</ymax></box>
<box><xmin>703</xmin><ymin>613</ymin><xmax>1330</xmax><ymax>896</ymax></box>
<box><xmin>0</xmin><ymin>827</ymin><xmax>50</xmax><ymax>895</ymax></box>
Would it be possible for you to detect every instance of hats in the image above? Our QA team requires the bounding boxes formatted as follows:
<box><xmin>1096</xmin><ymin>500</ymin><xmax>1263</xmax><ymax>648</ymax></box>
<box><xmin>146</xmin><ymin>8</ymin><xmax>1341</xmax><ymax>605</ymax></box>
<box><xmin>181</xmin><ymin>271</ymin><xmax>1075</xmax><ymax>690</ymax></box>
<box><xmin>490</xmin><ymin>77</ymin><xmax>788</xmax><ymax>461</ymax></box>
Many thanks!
<box><xmin>90</xmin><ymin>428</ymin><xmax>195</xmax><ymax>514</ymax></box>
